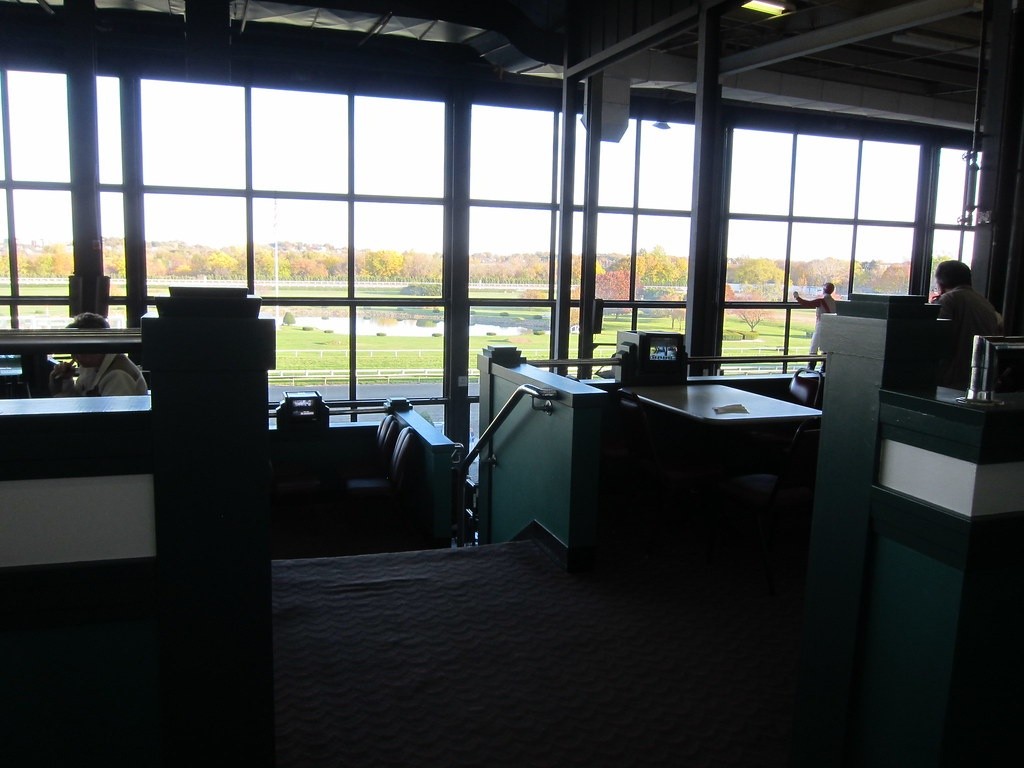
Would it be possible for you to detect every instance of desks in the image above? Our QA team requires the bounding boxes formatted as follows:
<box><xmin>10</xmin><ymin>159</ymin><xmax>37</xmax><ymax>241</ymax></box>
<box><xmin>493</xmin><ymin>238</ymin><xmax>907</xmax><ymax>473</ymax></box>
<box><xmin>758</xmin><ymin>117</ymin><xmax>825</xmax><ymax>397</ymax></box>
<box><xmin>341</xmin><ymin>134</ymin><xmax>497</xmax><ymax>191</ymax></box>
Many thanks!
<box><xmin>618</xmin><ymin>385</ymin><xmax>822</xmax><ymax>545</ymax></box>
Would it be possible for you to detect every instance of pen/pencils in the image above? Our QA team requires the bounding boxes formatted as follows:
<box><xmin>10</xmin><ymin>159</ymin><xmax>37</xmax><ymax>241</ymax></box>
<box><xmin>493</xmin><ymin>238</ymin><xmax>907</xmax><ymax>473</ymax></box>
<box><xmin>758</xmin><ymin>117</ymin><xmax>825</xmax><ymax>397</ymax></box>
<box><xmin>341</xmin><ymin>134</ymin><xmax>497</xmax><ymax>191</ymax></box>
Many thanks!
<box><xmin>55</xmin><ymin>366</ymin><xmax>76</xmax><ymax>380</ymax></box>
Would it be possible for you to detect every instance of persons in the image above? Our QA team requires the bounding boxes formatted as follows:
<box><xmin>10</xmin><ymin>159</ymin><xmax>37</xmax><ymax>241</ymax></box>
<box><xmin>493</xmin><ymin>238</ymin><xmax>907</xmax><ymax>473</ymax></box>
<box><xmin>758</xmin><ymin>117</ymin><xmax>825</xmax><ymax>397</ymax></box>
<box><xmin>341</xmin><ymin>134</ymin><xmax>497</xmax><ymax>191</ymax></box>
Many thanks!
<box><xmin>792</xmin><ymin>283</ymin><xmax>835</xmax><ymax>373</ymax></box>
<box><xmin>927</xmin><ymin>260</ymin><xmax>1004</xmax><ymax>392</ymax></box>
<box><xmin>48</xmin><ymin>313</ymin><xmax>148</xmax><ymax>396</ymax></box>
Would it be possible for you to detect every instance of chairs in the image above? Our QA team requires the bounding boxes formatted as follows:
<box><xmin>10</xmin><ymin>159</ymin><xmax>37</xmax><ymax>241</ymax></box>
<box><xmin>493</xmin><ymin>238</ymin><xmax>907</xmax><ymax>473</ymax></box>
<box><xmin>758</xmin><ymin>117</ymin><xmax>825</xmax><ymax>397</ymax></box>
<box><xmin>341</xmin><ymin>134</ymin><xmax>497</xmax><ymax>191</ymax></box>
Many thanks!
<box><xmin>329</xmin><ymin>414</ymin><xmax>415</xmax><ymax>546</ymax></box>
<box><xmin>566</xmin><ymin>367</ymin><xmax>823</xmax><ymax>612</ymax></box>
